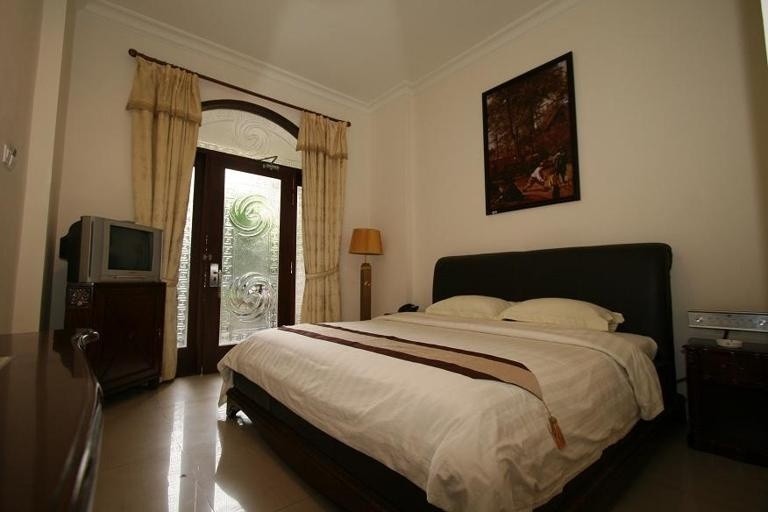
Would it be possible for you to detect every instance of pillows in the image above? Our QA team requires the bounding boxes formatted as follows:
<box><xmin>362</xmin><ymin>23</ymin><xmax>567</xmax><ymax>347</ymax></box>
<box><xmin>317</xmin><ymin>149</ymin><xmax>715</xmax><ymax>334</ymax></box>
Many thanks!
<box><xmin>424</xmin><ymin>292</ymin><xmax>510</xmax><ymax>322</ymax></box>
<box><xmin>494</xmin><ymin>296</ymin><xmax>625</xmax><ymax>333</ymax></box>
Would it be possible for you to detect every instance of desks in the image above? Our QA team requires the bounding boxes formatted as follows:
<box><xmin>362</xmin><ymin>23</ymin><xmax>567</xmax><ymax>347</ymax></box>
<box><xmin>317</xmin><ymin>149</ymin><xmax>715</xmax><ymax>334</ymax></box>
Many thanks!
<box><xmin>0</xmin><ymin>324</ymin><xmax>106</xmax><ymax>512</ymax></box>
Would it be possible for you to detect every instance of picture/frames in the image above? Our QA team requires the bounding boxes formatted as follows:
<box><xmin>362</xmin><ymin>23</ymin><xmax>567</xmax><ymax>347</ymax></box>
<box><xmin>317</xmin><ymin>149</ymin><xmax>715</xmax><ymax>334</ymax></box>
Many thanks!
<box><xmin>481</xmin><ymin>50</ymin><xmax>581</xmax><ymax>218</ymax></box>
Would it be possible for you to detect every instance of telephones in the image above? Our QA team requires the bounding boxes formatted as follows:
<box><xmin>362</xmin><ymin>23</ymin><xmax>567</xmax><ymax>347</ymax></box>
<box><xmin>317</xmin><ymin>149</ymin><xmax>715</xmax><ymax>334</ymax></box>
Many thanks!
<box><xmin>398</xmin><ymin>303</ymin><xmax>419</xmax><ymax>312</ymax></box>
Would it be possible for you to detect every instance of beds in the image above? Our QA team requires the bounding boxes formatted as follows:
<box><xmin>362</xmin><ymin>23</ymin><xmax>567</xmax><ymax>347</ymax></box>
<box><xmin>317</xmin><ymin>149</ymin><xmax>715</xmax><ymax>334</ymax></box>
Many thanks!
<box><xmin>216</xmin><ymin>242</ymin><xmax>675</xmax><ymax>512</ymax></box>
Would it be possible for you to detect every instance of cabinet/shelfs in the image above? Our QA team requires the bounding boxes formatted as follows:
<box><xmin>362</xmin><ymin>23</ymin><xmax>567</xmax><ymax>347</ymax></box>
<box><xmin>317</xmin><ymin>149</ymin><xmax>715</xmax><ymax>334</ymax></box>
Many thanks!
<box><xmin>65</xmin><ymin>281</ymin><xmax>167</xmax><ymax>409</ymax></box>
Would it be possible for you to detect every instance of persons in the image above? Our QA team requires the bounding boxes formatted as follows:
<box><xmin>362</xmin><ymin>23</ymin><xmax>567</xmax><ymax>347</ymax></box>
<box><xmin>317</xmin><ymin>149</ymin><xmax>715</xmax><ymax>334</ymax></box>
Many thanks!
<box><xmin>494</xmin><ymin>146</ymin><xmax>568</xmax><ymax>204</ymax></box>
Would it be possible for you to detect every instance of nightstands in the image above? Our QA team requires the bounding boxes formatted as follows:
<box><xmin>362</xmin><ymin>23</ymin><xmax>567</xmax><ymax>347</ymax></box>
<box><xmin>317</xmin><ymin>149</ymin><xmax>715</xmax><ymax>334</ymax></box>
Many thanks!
<box><xmin>681</xmin><ymin>337</ymin><xmax>768</xmax><ymax>465</ymax></box>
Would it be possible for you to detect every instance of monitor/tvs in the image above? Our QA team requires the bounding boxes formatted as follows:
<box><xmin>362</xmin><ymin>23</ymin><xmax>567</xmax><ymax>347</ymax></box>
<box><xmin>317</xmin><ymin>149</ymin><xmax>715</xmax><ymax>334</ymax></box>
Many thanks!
<box><xmin>59</xmin><ymin>215</ymin><xmax>162</xmax><ymax>283</ymax></box>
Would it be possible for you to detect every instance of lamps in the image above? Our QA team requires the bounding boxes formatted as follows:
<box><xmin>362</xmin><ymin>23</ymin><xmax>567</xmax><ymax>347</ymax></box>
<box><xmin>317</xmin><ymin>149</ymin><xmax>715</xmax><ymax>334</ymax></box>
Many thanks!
<box><xmin>347</xmin><ymin>227</ymin><xmax>384</xmax><ymax>320</ymax></box>
